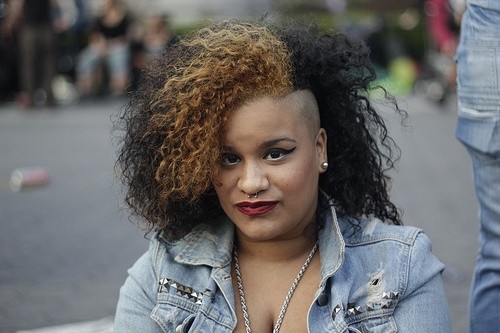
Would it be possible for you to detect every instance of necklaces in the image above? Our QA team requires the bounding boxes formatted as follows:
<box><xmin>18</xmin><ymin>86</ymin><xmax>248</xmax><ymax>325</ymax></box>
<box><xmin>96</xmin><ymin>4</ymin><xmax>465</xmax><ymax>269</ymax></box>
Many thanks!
<box><xmin>232</xmin><ymin>242</ymin><xmax>319</xmax><ymax>333</ymax></box>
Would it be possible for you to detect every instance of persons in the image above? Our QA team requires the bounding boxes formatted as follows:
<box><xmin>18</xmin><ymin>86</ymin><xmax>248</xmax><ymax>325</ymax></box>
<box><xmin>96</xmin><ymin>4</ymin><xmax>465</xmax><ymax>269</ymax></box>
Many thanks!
<box><xmin>110</xmin><ymin>12</ymin><xmax>454</xmax><ymax>333</ymax></box>
<box><xmin>0</xmin><ymin>0</ymin><xmax>461</xmax><ymax>110</ymax></box>
<box><xmin>455</xmin><ymin>0</ymin><xmax>500</xmax><ymax>332</ymax></box>
